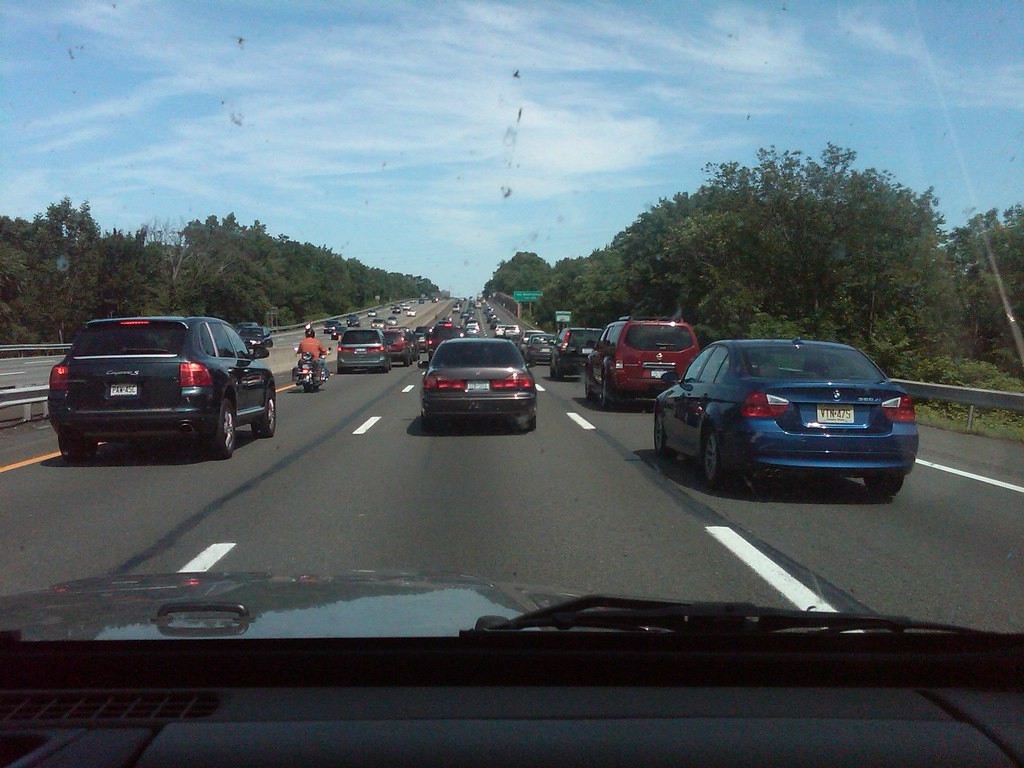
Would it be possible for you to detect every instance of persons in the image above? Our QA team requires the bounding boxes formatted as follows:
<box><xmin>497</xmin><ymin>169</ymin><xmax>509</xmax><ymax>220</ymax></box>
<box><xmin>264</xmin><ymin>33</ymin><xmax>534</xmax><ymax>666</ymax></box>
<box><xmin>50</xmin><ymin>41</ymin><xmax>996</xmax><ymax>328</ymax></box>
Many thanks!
<box><xmin>294</xmin><ymin>330</ymin><xmax>330</xmax><ymax>382</ymax></box>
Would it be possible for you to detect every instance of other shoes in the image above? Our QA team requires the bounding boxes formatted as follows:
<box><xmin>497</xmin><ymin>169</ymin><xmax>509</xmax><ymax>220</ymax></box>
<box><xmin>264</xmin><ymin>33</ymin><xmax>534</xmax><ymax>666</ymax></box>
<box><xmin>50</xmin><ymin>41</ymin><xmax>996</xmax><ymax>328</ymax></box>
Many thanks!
<box><xmin>321</xmin><ymin>376</ymin><xmax>329</xmax><ymax>381</ymax></box>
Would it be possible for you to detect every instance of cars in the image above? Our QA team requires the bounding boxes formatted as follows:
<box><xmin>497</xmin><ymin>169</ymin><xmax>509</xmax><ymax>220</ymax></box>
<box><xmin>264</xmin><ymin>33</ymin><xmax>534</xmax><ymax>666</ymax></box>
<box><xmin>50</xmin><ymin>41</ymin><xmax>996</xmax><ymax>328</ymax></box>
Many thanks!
<box><xmin>418</xmin><ymin>338</ymin><xmax>540</xmax><ymax>432</ymax></box>
<box><xmin>371</xmin><ymin>317</ymin><xmax>388</xmax><ymax>329</ymax></box>
<box><xmin>511</xmin><ymin>334</ymin><xmax>522</xmax><ymax>346</ymax></box>
<box><xmin>368</xmin><ymin>309</ymin><xmax>378</xmax><ymax>317</ymax></box>
<box><xmin>235</xmin><ymin>322</ymin><xmax>257</xmax><ymax>333</ymax></box>
<box><xmin>337</xmin><ymin>328</ymin><xmax>393</xmax><ymax>373</ymax></box>
<box><xmin>503</xmin><ymin>324</ymin><xmax>520</xmax><ymax>337</ymax></box>
<box><xmin>324</xmin><ymin>313</ymin><xmax>361</xmax><ymax>339</ymax></box>
<box><xmin>518</xmin><ymin>329</ymin><xmax>546</xmax><ymax>357</ymax></box>
<box><xmin>495</xmin><ymin>325</ymin><xmax>508</xmax><ymax>338</ymax></box>
<box><xmin>382</xmin><ymin>292</ymin><xmax>503</xmax><ymax>361</ymax></box>
<box><xmin>651</xmin><ymin>336</ymin><xmax>919</xmax><ymax>499</ymax></box>
<box><xmin>381</xmin><ymin>330</ymin><xmax>415</xmax><ymax>367</ymax></box>
<box><xmin>387</xmin><ymin>315</ymin><xmax>401</xmax><ymax>326</ymax></box>
<box><xmin>522</xmin><ymin>334</ymin><xmax>558</xmax><ymax>366</ymax></box>
<box><xmin>238</xmin><ymin>327</ymin><xmax>273</xmax><ymax>348</ymax></box>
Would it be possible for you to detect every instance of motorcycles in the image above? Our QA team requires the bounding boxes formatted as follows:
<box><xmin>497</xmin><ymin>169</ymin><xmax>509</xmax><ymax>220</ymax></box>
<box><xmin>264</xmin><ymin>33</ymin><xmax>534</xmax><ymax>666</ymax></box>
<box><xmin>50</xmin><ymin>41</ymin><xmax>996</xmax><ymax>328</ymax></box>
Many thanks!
<box><xmin>293</xmin><ymin>347</ymin><xmax>333</xmax><ymax>392</ymax></box>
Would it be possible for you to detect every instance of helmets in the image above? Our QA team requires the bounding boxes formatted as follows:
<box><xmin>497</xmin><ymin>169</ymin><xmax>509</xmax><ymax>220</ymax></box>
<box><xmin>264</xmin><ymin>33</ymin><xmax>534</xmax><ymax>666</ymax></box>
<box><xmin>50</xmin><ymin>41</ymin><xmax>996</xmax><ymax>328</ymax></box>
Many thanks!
<box><xmin>305</xmin><ymin>328</ymin><xmax>315</xmax><ymax>338</ymax></box>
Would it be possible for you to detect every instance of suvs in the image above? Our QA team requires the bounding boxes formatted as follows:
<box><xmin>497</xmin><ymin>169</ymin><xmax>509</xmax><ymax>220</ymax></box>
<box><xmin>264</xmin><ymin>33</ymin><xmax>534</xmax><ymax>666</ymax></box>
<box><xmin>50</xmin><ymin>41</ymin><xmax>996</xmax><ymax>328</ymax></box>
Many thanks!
<box><xmin>46</xmin><ymin>313</ymin><xmax>278</xmax><ymax>460</ymax></box>
<box><xmin>583</xmin><ymin>317</ymin><xmax>700</xmax><ymax>411</ymax></box>
<box><xmin>425</xmin><ymin>322</ymin><xmax>471</xmax><ymax>364</ymax></box>
<box><xmin>549</xmin><ymin>327</ymin><xmax>608</xmax><ymax>381</ymax></box>
